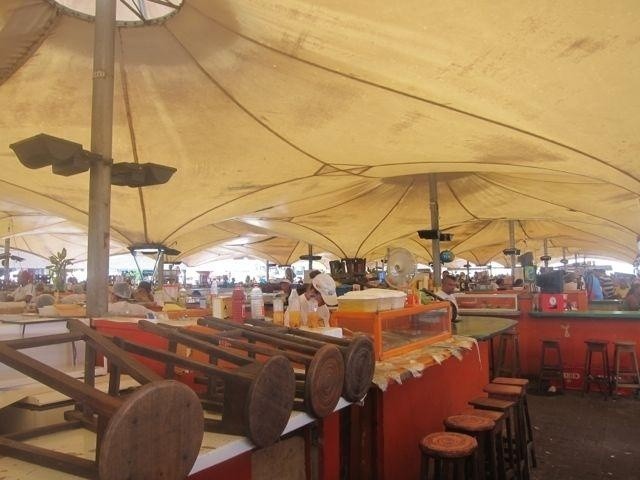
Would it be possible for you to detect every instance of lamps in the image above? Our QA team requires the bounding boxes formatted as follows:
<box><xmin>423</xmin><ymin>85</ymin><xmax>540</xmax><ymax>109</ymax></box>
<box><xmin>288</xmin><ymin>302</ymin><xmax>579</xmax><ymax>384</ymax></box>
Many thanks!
<box><xmin>7</xmin><ymin>132</ymin><xmax>177</xmax><ymax>188</ymax></box>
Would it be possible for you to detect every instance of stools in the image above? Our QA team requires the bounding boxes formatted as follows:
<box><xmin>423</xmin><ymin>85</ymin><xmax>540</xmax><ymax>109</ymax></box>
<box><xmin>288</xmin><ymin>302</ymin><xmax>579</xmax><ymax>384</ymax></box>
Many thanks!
<box><xmin>497</xmin><ymin>330</ymin><xmax>640</xmax><ymax>401</ymax></box>
<box><xmin>417</xmin><ymin>374</ymin><xmax>539</xmax><ymax>479</ymax></box>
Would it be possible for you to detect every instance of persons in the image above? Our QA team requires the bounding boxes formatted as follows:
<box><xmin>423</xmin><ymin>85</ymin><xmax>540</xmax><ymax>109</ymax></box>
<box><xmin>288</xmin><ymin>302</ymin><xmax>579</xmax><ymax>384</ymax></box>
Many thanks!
<box><xmin>6</xmin><ymin>265</ymin><xmax>640</xmax><ymax>327</ymax></box>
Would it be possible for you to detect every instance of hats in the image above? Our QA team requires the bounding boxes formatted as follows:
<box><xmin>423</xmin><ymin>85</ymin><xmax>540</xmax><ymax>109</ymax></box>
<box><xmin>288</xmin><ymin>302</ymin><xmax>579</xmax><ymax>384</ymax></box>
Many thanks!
<box><xmin>312</xmin><ymin>273</ymin><xmax>338</xmax><ymax>306</ymax></box>
<box><xmin>281</xmin><ymin>279</ymin><xmax>291</xmax><ymax>284</ymax></box>
<box><xmin>303</xmin><ymin>270</ymin><xmax>317</xmax><ymax>285</ymax></box>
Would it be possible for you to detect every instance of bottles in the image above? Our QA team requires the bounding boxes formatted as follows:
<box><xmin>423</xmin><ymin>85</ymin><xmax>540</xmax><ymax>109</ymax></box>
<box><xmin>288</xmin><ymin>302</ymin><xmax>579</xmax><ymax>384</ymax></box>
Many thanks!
<box><xmin>231</xmin><ymin>287</ymin><xmax>246</xmax><ymax>324</ymax></box>
<box><xmin>272</xmin><ymin>293</ymin><xmax>283</xmax><ymax>326</ymax></box>
<box><xmin>307</xmin><ymin>294</ymin><xmax>318</xmax><ymax>328</ymax></box>
<box><xmin>406</xmin><ymin>290</ymin><xmax>414</xmax><ymax>306</ymax></box>
<box><xmin>210</xmin><ymin>278</ymin><xmax>218</xmax><ymax>294</ymax></box>
<box><xmin>250</xmin><ymin>284</ymin><xmax>265</xmax><ymax>319</ymax></box>
<box><xmin>287</xmin><ymin>285</ymin><xmax>301</xmax><ymax>328</ymax></box>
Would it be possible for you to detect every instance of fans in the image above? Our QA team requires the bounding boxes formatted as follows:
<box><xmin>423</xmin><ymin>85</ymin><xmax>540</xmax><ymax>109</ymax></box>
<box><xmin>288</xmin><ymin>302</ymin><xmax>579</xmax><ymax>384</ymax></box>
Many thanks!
<box><xmin>381</xmin><ymin>248</ymin><xmax>418</xmax><ymax>291</ymax></box>
